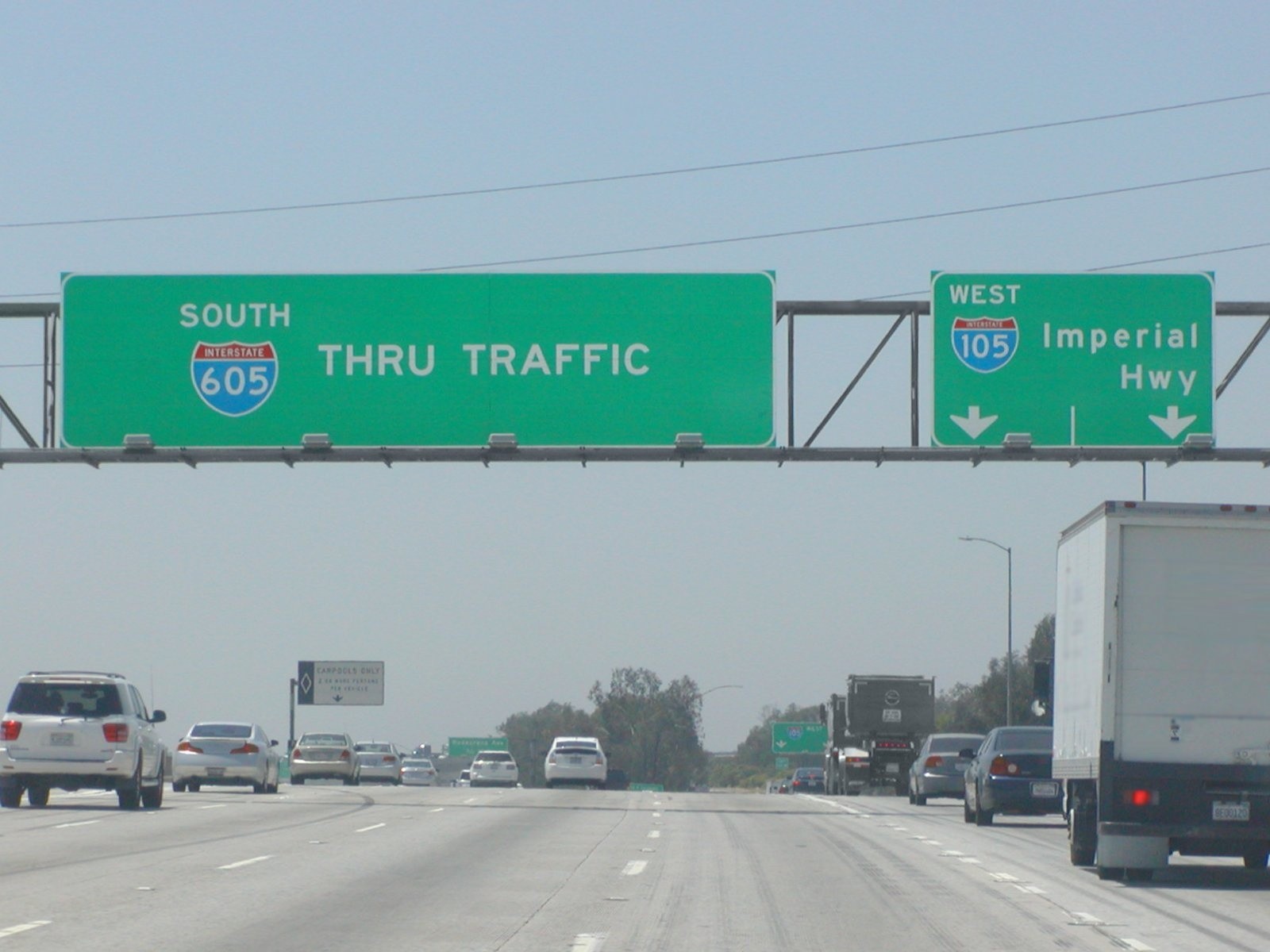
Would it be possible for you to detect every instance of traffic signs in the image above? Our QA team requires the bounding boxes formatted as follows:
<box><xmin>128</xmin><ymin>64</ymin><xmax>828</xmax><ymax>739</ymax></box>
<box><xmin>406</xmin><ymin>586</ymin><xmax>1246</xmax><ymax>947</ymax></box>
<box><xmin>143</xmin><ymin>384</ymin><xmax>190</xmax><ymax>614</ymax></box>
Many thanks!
<box><xmin>297</xmin><ymin>660</ymin><xmax>384</xmax><ymax>705</ymax></box>
<box><xmin>771</xmin><ymin>722</ymin><xmax>828</xmax><ymax>754</ymax></box>
<box><xmin>928</xmin><ymin>268</ymin><xmax>1215</xmax><ymax>449</ymax></box>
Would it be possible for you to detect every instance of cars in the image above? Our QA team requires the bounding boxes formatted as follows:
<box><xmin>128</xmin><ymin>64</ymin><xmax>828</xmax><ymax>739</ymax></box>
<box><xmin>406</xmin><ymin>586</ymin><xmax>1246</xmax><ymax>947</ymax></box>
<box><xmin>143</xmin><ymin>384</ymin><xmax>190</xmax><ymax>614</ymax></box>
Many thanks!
<box><xmin>787</xmin><ymin>766</ymin><xmax>826</xmax><ymax>794</ymax></box>
<box><xmin>769</xmin><ymin>778</ymin><xmax>791</xmax><ymax>794</ymax></box>
<box><xmin>450</xmin><ymin>769</ymin><xmax>471</xmax><ymax>788</ymax></box>
<box><xmin>171</xmin><ymin>721</ymin><xmax>282</xmax><ymax>795</ymax></box>
<box><xmin>398</xmin><ymin>758</ymin><xmax>440</xmax><ymax>787</ymax></box>
<box><xmin>908</xmin><ymin>732</ymin><xmax>986</xmax><ymax>805</ymax></box>
<box><xmin>542</xmin><ymin>735</ymin><xmax>611</xmax><ymax>790</ymax></box>
<box><xmin>353</xmin><ymin>740</ymin><xmax>407</xmax><ymax>785</ymax></box>
<box><xmin>467</xmin><ymin>750</ymin><xmax>520</xmax><ymax>788</ymax></box>
<box><xmin>288</xmin><ymin>730</ymin><xmax>362</xmax><ymax>786</ymax></box>
<box><xmin>958</xmin><ymin>725</ymin><xmax>1066</xmax><ymax>826</ymax></box>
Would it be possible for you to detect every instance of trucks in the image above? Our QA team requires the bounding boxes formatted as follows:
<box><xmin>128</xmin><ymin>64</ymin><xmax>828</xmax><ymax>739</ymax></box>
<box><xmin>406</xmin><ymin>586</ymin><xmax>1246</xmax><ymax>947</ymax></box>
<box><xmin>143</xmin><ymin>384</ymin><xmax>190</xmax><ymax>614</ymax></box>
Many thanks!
<box><xmin>823</xmin><ymin>674</ymin><xmax>936</xmax><ymax>797</ymax></box>
<box><xmin>1033</xmin><ymin>499</ymin><xmax>1269</xmax><ymax>884</ymax></box>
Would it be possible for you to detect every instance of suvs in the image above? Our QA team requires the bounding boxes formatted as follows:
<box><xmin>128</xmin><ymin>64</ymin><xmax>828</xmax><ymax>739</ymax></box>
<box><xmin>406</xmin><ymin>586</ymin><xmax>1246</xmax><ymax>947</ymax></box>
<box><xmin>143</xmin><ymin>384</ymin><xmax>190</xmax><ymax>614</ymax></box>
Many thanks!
<box><xmin>1</xmin><ymin>669</ymin><xmax>169</xmax><ymax>811</ymax></box>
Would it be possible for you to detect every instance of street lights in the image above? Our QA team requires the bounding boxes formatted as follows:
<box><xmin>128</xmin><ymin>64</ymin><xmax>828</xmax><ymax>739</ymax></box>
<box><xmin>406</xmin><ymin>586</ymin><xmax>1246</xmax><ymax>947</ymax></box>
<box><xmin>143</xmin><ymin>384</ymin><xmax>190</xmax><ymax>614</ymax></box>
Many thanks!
<box><xmin>958</xmin><ymin>536</ymin><xmax>1013</xmax><ymax>726</ymax></box>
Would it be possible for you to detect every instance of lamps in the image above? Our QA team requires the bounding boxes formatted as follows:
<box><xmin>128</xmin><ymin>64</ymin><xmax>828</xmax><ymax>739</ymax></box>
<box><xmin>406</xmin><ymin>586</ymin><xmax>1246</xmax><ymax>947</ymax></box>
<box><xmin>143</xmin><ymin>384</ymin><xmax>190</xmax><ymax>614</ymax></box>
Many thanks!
<box><xmin>1001</xmin><ymin>433</ymin><xmax>1032</xmax><ymax>448</ymax></box>
<box><xmin>487</xmin><ymin>434</ymin><xmax>518</xmax><ymax>449</ymax></box>
<box><xmin>675</xmin><ymin>432</ymin><xmax>704</xmax><ymax>448</ymax></box>
<box><xmin>122</xmin><ymin>434</ymin><xmax>156</xmax><ymax>451</ymax></box>
<box><xmin>300</xmin><ymin>434</ymin><xmax>333</xmax><ymax>449</ymax></box>
<box><xmin>1182</xmin><ymin>434</ymin><xmax>1213</xmax><ymax>449</ymax></box>
